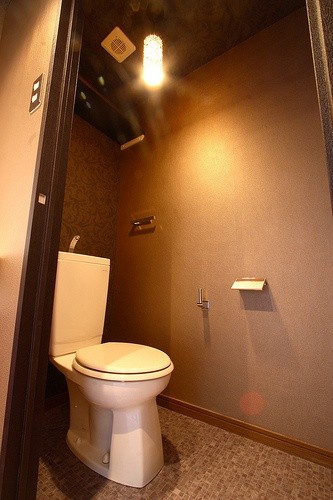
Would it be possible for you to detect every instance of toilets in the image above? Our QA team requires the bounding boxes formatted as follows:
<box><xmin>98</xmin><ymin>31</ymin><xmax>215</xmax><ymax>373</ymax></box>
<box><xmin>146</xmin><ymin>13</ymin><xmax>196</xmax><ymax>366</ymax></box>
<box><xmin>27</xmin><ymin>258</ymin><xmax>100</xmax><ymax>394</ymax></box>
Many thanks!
<box><xmin>49</xmin><ymin>250</ymin><xmax>175</xmax><ymax>489</ymax></box>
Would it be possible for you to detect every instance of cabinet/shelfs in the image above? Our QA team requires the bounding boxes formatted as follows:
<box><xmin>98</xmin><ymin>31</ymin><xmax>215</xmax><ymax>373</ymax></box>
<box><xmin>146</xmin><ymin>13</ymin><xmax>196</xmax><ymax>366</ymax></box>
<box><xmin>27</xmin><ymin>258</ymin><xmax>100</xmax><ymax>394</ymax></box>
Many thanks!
<box><xmin>74</xmin><ymin>75</ymin><xmax>144</xmax><ymax>151</ymax></box>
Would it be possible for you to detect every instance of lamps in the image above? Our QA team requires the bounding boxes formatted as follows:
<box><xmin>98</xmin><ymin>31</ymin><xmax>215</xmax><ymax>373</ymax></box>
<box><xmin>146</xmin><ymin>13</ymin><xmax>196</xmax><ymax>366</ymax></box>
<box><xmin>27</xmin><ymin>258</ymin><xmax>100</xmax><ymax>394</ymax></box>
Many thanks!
<box><xmin>139</xmin><ymin>34</ymin><xmax>167</xmax><ymax>88</ymax></box>
